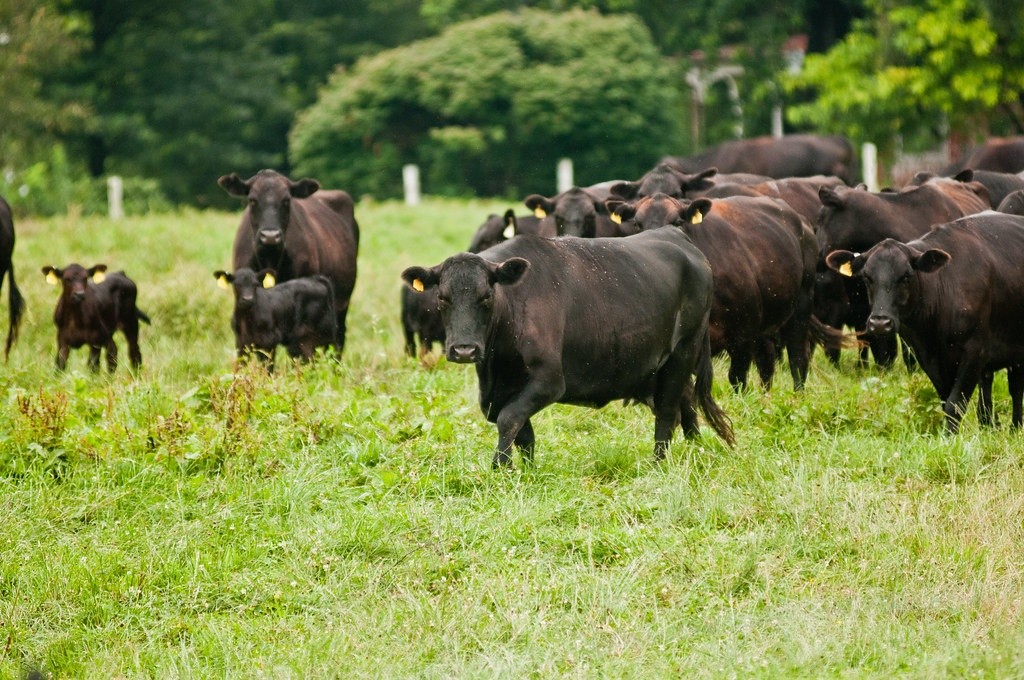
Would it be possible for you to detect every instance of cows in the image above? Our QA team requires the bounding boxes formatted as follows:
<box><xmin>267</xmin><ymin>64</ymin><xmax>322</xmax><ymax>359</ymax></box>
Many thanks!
<box><xmin>0</xmin><ymin>193</ymin><xmax>27</xmax><ymax>364</ymax></box>
<box><xmin>398</xmin><ymin>132</ymin><xmax>1024</xmax><ymax>475</ymax></box>
<box><xmin>212</xmin><ymin>168</ymin><xmax>360</xmax><ymax>379</ymax></box>
<box><xmin>40</xmin><ymin>261</ymin><xmax>152</xmax><ymax>377</ymax></box>
